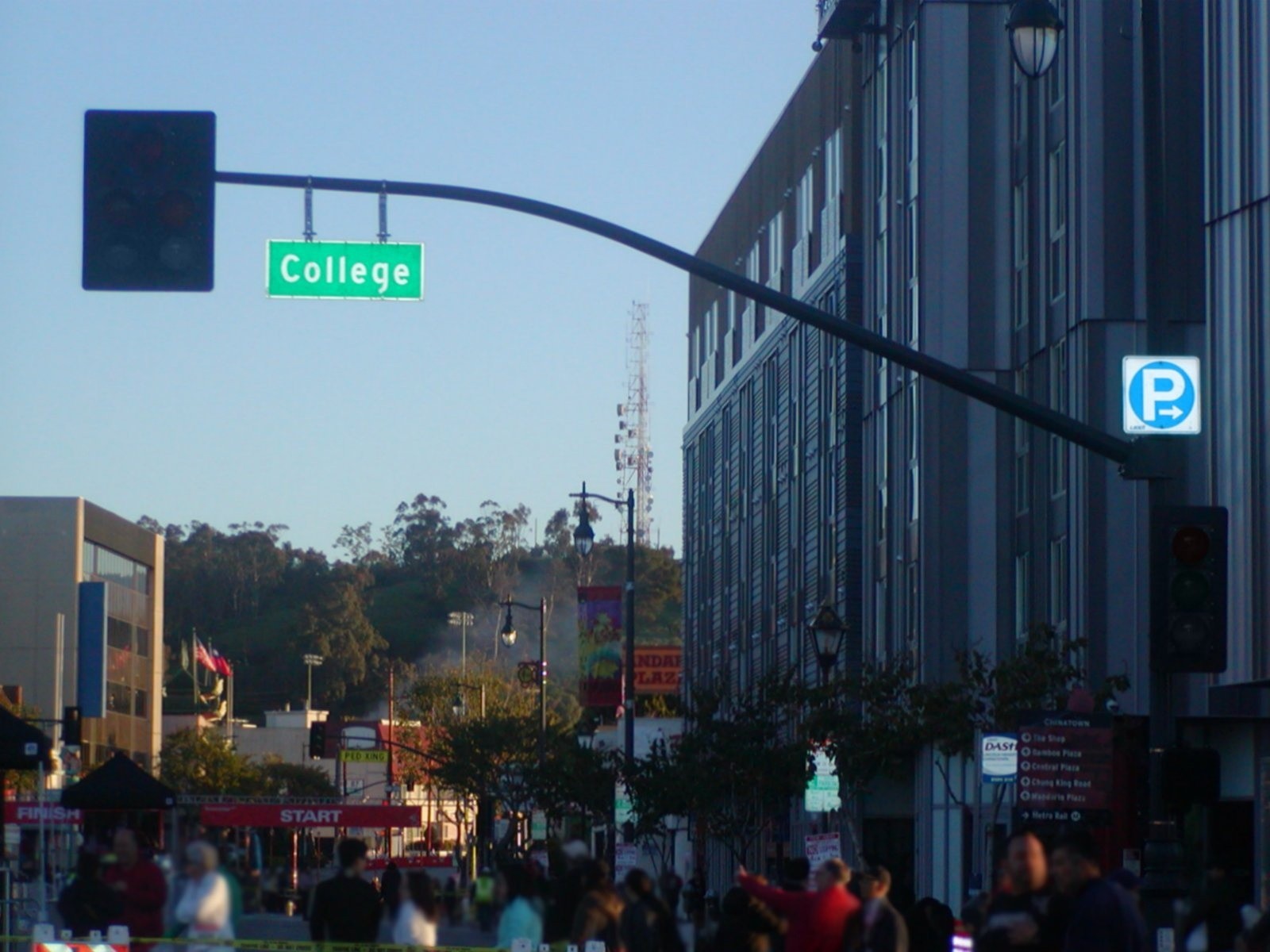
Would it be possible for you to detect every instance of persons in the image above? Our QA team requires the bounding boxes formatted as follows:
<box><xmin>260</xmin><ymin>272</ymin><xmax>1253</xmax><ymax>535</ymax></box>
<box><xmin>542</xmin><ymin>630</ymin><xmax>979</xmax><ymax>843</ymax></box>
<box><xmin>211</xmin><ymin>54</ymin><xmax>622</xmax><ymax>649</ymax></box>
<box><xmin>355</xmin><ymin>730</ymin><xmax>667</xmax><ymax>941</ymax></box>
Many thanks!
<box><xmin>55</xmin><ymin>820</ymin><xmax>1270</xmax><ymax>952</ymax></box>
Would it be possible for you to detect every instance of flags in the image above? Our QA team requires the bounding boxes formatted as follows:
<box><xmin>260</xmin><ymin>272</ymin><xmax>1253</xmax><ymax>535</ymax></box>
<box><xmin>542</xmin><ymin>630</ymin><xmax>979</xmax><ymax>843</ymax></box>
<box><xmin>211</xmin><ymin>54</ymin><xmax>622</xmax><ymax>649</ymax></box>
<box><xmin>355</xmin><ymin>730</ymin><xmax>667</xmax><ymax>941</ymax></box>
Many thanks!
<box><xmin>210</xmin><ymin>645</ymin><xmax>234</xmax><ymax>678</ymax></box>
<box><xmin>194</xmin><ymin>637</ymin><xmax>218</xmax><ymax>670</ymax></box>
<box><xmin>576</xmin><ymin>585</ymin><xmax>623</xmax><ymax>706</ymax></box>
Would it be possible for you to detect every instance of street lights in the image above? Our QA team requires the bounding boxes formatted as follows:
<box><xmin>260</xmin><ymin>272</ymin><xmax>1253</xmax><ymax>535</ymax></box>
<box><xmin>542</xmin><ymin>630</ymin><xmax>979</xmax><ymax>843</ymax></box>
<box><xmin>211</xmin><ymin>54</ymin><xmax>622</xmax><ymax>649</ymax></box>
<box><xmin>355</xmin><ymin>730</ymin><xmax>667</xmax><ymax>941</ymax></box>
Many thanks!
<box><xmin>999</xmin><ymin>1</ymin><xmax>1066</xmax><ymax>661</ymax></box>
<box><xmin>449</xmin><ymin>611</ymin><xmax>475</xmax><ymax>678</ymax></box>
<box><xmin>304</xmin><ymin>652</ymin><xmax>323</xmax><ymax>710</ymax></box>
<box><xmin>569</xmin><ymin>481</ymin><xmax>636</xmax><ymax>786</ymax></box>
<box><xmin>496</xmin><ymin>593</ymin><xmax>548</xmax><ymax>766</ymax></box>
<box><xmin>804</xmin><ymin>603</ymin><xmax>839</xmax><ymax>832</ymax></box>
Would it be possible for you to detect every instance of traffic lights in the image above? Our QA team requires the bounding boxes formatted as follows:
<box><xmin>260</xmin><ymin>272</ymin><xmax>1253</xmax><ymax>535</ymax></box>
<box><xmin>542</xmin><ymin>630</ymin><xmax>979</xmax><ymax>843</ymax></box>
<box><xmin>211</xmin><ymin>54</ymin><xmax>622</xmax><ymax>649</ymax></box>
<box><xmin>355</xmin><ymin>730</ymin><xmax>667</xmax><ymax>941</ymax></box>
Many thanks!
<box><xmin>1149</xmin><ymin>504</ymin><xmax>1229</xmax><ymax>674</ymax></box>
<box><xmin>83</xmin><ymin>110</ymin><xmax>215</xmax><ymax>293</ymax></box>
<box><xmin>62</xmin><ymin>707</ymin><xmax>82</xmax><ymax>744</ymax></box>
